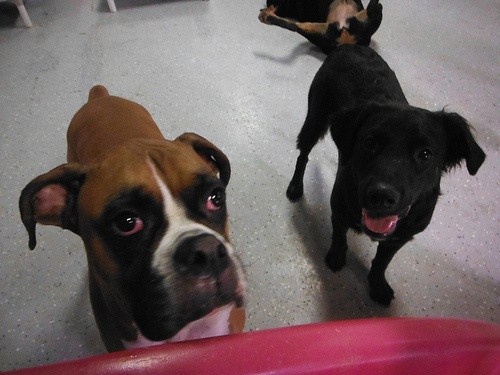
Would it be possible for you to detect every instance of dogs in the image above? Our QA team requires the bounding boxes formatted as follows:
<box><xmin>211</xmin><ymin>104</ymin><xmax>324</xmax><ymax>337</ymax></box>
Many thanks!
<box><xmin>286</xmin><ymin>44</ymin><xmax>487</xmax><ymax>307</ymax></box>
<box><xmin>19</xmin><ymin>83</ymin><xmax>249</xmax><ymax>354</ymax></box>
<box><xmin>258</xmin><ymin>0</ymin><xmax>383</xmax><ymax>56</ymax></box>
